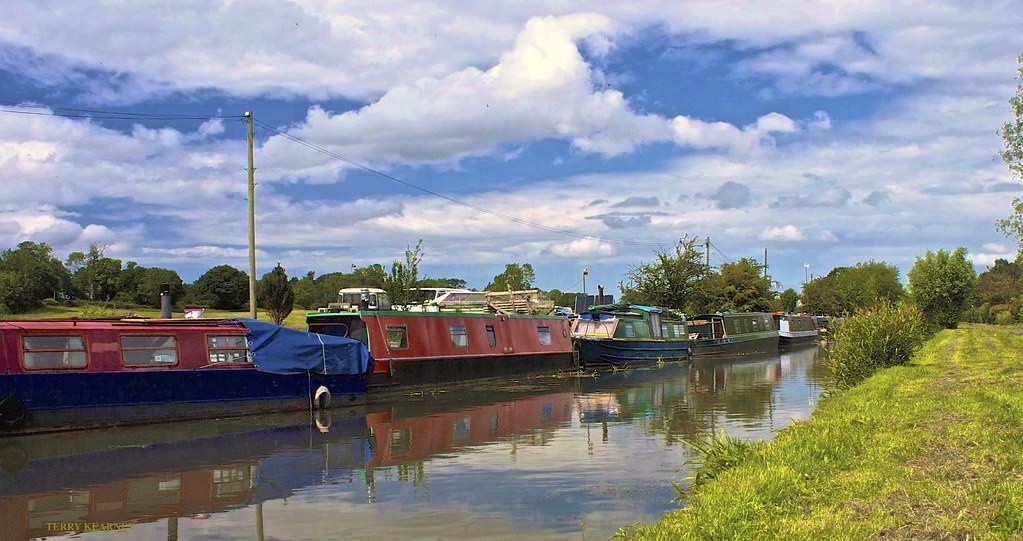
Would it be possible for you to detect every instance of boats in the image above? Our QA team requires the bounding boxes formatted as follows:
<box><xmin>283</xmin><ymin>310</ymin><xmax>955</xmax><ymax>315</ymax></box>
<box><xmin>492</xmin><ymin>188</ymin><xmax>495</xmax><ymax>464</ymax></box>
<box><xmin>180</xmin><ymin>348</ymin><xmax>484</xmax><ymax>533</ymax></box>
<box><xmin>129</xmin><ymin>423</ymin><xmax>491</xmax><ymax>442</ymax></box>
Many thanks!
<box><xmin>570</xmin><ymin>284</ymin><xmax>691</xmax><ymax>364</ymax></box>
<box><xmin>0</xmin><ymin>316</ymin><xmax>376</xmax><ymax>429</ymax></box>
<box><xmin>307</xmin><ymin>309</ymin><xmax>582</xmax><ymax>394</ymax></box>
<box><xmin>779</xmin><ymin>313</ymin><xmax>837</xmax><ymax>340</ymax></box>
<box><xmin>679</xmin><ymin>312</ymin><xmax>778</xmax><ymax>348</ymax></box>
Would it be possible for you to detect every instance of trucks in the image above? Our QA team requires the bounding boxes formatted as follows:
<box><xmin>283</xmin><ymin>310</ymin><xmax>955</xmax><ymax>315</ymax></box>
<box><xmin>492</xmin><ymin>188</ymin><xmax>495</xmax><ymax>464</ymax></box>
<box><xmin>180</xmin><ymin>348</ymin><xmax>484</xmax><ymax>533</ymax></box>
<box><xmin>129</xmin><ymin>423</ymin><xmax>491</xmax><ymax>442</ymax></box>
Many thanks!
<box><xmin>319</xmin><ymin>287</ymin><xmax>538</xmax><ymax>314</ymax></box>
